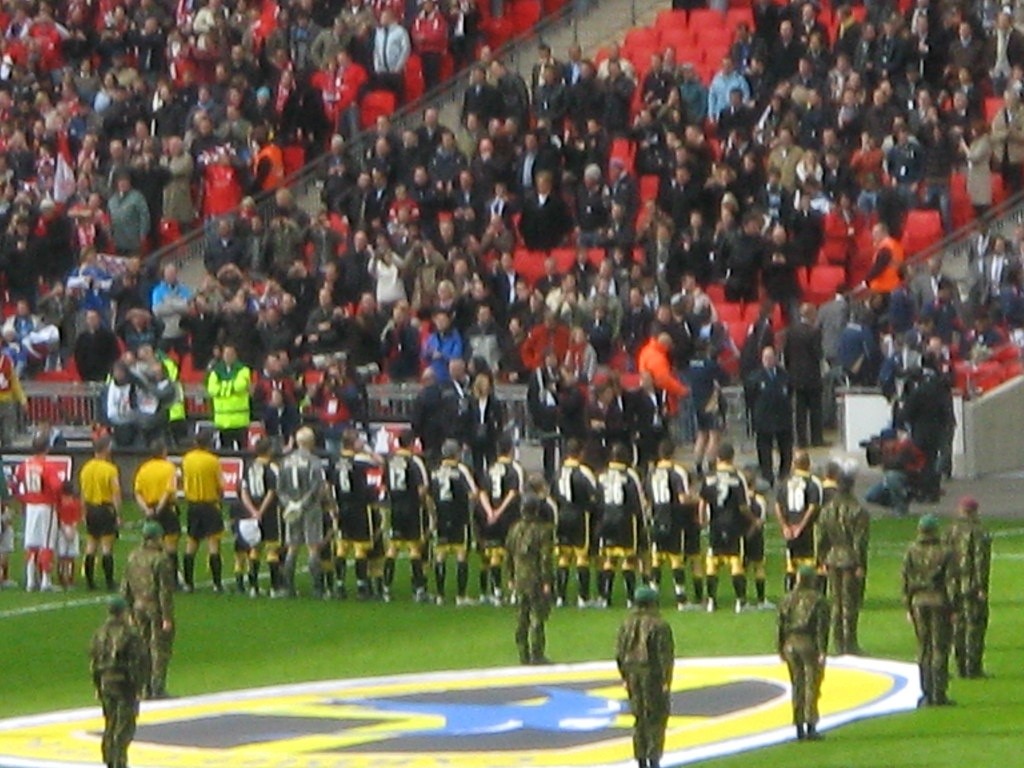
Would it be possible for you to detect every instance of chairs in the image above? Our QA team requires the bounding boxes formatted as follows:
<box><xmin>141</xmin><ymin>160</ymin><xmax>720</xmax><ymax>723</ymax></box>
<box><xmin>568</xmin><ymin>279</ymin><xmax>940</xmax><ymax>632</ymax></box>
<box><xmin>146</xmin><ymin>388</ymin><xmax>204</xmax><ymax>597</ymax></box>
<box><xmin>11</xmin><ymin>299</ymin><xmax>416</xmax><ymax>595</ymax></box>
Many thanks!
<box><xmin>33</xmin><ymin>0</ymin><xmax>1022</xmax><ymax>423</ymax></box>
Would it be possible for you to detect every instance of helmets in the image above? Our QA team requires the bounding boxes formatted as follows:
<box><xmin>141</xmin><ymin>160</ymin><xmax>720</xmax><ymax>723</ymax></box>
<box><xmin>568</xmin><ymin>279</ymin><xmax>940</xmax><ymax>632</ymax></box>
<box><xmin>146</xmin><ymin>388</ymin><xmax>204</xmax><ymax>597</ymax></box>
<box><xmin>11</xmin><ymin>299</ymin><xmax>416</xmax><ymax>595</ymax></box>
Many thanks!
<box><xmin>634</xmin><ymin>588</ymin><xmax>657</xmax><ymax>606</ymax></box>
<box><xmin>142</xmin><ymin>521</ymin><xmax>165</xmax><ymax>539</ymax></box>
<box><xmin>799</xmin><ymin>566</ymin><xmax>818</xmax><ymax>575</ymax></box>
<box><xmin>520</xmin><ymin>494</ymin><xmax>542</xmax><ymax>517</ymax></box>
<box><xmin>917</xmin><ymin>514</ymin><xmax>940</xmax><ymax>535</ymax></box>
<box><xmin>105</xmin><ymin>593</ymin><xmax>131</xmax><ymax>611</ymax></box>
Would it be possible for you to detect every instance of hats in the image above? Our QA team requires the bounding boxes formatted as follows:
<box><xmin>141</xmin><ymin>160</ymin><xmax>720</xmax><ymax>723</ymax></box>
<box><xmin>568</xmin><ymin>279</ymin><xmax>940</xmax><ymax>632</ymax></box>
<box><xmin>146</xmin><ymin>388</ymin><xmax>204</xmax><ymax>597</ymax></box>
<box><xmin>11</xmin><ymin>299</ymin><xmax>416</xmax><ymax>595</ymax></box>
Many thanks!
<box><xmin>959</xmin><ymin>498</ymin><xmax>978</xmax><ymax>510</ymax></box>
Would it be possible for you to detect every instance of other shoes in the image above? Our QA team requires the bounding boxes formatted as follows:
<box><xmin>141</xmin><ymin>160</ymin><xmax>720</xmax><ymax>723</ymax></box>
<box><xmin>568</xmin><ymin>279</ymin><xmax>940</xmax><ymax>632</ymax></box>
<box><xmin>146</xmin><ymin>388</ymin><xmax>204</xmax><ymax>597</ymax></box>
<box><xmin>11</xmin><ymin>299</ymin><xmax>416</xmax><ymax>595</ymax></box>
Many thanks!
<box><xmin>178</xmin><ymin>582</ymin><xmax>519</xmax><ymax>605</ymax></box>
<box><xmin>797</xmin><ymin>723</ymin><xmax>825</xmax><ymax>741</ymax></box>
<box><xmin>8</xmin><ymin>579</ymin><xmax>122</xmax><ymax>591</ymax></box>
<box><xmin>639</xmin><ymin>758</ymin><xmax>661</xmax><ymax>768</ymax></box>
<box><xmin>918</xmin><ymin>691</ymin><xmax>951</xmax><ymax>706</ymax></box>
<box><xmin>140</xmin><ymin>691</ymin><xmax>167</xmax><ymax>699</ymax></box>
<box><xmin>552</xmin><ymin>596</ymin><xmax>780</xmax><ymax>612</ymax></box>
<box><xmin>522</xmin><ymin>656</ymin><xmax>551</xmax><ymax>664</ymax></box>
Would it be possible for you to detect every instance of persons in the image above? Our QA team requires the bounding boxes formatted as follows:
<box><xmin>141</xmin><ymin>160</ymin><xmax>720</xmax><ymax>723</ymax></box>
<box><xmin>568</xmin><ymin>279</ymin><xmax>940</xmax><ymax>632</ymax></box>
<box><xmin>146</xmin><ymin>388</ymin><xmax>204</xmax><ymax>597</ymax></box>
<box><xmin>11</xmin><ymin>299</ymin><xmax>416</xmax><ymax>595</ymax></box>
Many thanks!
<box><xmin>900</xmin><ymin>514</ymin><xmax>962</xmax><ymax>707</ymax></box>
<box><xmin>813</xmin><ymin>475</ymin><xmax>870</xmax><ymax>657</ymax></box>
<box><xmin>941</xmin><ymin>498</ymin><xmax>992</xmax><ymax>678</ymax></box>
<box><xmin>615</xmin><ymin>587</ymin><xmax>676</xmax><ymax>768</ymax></box>
<box><xmin>500</xmin><ymin>494</ymin><xmax>557</xmax><ymax>665</ymax></box>
<box><xmin>89</xmin><ymin>594</ymin><xmax>147</xmax><ymax>768</ymax></box>
<box><xmin>120</xmin><ymin>520</ymin><xmax>176</xmax><ymax>700</ymax></box>
<box><xmin>0</xmin><ymin>0</ymin><xmax>1024</xmax><ymax>614</ymax></box>
<box><xmin>777</xmin><ymin>566</ymin><xmax>833</xmax><ymax>740</ymax></box>
